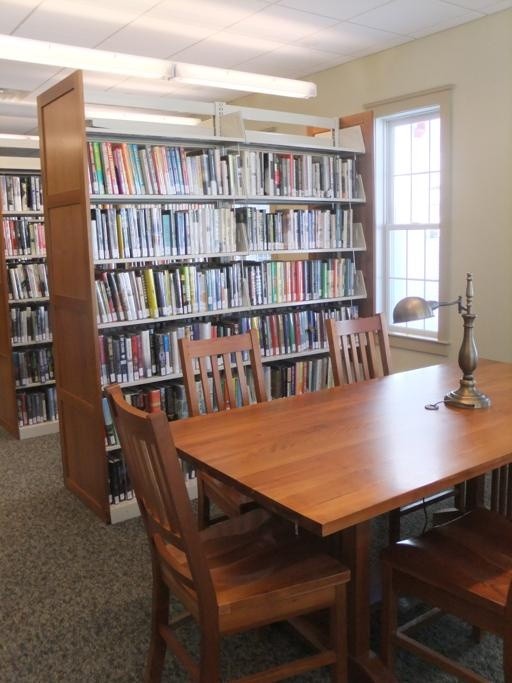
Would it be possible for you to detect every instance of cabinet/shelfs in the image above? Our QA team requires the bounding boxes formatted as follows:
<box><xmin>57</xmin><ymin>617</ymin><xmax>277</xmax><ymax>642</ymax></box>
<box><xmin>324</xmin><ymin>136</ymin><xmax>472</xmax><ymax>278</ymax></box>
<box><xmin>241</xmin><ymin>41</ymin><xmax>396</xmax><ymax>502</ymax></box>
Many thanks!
<box><xmin>1</xmin><ymin>164</ymin><xmax>58</xmax><ymax>443</ymax></box>
<box><xmin>37</xmin><ymin>67</ymin><xmax>383</xmax><ymax>526</ymax></box>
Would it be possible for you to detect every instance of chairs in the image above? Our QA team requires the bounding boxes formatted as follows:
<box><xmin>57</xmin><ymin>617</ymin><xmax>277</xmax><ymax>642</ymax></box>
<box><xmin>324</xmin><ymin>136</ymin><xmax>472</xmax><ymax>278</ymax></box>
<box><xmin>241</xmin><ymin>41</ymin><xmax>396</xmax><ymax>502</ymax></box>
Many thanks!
<box><xmin>172</xmin><ymin>330</ymin><xmax>287</xmax><ymax>531</ymax></box>
<box><xmin>382</xmin><ymin>507</ymin><xmax>512</xmax><ymax>682</ymax></box>
<box><xmin>104</xmin><ymin>382</ymin><xmax>354</xmax><ymax>681</ymax></box>
<box><xmin>322</xmin><ymin>314</ymin><xmax>465</xmax><ymax>541</ymax></box>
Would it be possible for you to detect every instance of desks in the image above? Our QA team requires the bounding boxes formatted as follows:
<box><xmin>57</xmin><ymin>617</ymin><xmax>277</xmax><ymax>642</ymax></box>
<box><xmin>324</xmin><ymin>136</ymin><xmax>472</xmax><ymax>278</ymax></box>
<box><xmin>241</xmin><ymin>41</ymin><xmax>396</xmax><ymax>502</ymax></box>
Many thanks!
<box><xmin>164</xmin><ymin>355</ymin><xmax>511</xmax><ymax>679</ymax></box>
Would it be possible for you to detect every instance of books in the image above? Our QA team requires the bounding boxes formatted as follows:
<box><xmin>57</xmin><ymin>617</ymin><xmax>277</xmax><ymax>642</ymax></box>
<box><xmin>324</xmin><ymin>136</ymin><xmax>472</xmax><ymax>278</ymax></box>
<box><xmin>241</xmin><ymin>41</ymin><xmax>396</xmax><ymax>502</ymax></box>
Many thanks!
<box><xmin>94</xmin><ymin>258</ymin><xmax>357</xmax><ymax>325</ymax></box>
<box><xmin>0</xmin><ymin>168</ymin><xmax>61</xmax><ymax>429</ymax></box>
<box><xmin>90</xmin><ymin>204</ymin><xmax>352</xmax><ymax>260</ymax></box>
<box><xmin>98</xmin><ymin>304</ymin><xmax>360</xmax><ymax>386</ymax></box>
<box><xmin>87</xmin><ymin>142</ymin><xmax>365</xmax><ymax>197</ymax></box>
<box><xmin>102</xmin><ymin>353</ymin><xmax>345</xmax><ymax>504</ymax></box>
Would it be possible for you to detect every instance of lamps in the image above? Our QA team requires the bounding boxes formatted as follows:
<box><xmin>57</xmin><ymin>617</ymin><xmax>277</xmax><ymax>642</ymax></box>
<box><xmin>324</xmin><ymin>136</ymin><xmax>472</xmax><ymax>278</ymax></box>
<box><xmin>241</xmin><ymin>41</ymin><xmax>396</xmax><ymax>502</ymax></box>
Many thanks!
<box><xmin>391</xmin><ymin>271</ymin><xmax>493</xmax><ymax>410</ymax></box>
